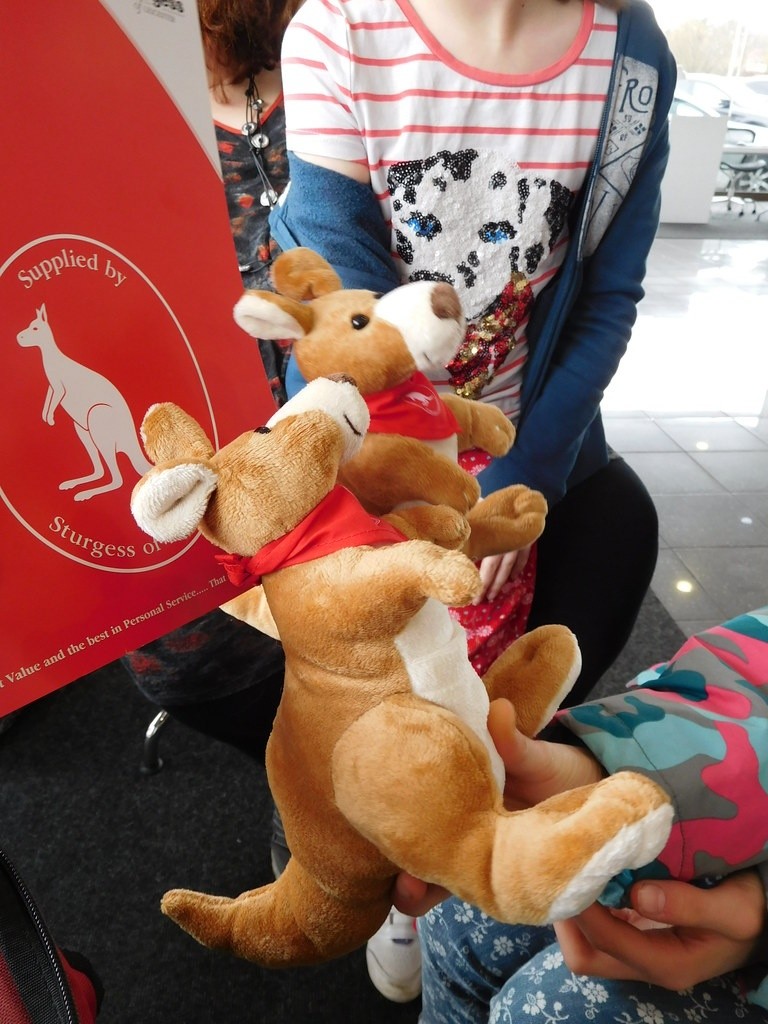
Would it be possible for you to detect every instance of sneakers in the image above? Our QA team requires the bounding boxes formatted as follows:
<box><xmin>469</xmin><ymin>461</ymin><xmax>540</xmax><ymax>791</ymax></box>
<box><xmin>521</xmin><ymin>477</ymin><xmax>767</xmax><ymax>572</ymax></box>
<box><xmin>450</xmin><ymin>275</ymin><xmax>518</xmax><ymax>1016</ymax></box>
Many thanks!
<box><xmin>365</xmin><ymin>910</ymin><xmax>423</xmax><ymax>1003</ymax></box>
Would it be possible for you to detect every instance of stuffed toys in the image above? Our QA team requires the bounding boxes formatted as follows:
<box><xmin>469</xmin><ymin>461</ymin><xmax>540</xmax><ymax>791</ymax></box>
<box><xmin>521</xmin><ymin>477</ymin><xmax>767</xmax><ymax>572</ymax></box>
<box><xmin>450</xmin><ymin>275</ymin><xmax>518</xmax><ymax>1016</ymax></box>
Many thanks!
<box><xmin>128</xmin><ymin>246</ymin><xmax>673</xmax><ymax>972</ymax></box>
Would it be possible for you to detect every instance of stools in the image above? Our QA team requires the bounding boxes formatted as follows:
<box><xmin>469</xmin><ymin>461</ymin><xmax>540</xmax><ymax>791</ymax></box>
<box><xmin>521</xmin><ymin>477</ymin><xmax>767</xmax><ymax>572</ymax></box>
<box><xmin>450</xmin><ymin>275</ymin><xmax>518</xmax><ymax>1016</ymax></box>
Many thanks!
<box><xmin>116</xmin><ymin>603</ymin><xmax>284</xmax><ymax>780</ymax></box>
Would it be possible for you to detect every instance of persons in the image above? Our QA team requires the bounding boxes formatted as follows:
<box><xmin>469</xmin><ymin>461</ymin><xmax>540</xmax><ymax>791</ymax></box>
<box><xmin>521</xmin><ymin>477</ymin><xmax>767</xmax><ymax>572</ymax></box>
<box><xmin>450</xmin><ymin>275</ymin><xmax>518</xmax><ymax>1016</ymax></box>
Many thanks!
<box><xmin>201</xmin><ymin>1</ymin><xmax>675</xmax><ymax>1002</ymax></box>
<box><xmin>413</xmin><ymin>604</ymin><xmax>768</xmax><ymax>1023</ymax></box>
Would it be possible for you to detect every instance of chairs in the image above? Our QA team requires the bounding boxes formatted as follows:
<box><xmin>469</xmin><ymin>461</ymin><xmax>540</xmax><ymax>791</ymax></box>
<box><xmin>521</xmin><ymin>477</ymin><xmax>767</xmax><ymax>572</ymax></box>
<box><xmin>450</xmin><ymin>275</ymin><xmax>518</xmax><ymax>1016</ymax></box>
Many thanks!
<box><xmin>719</xmin><ymin>128</ymin><xmax>765</xmax><ymax>217</ymax></box>
